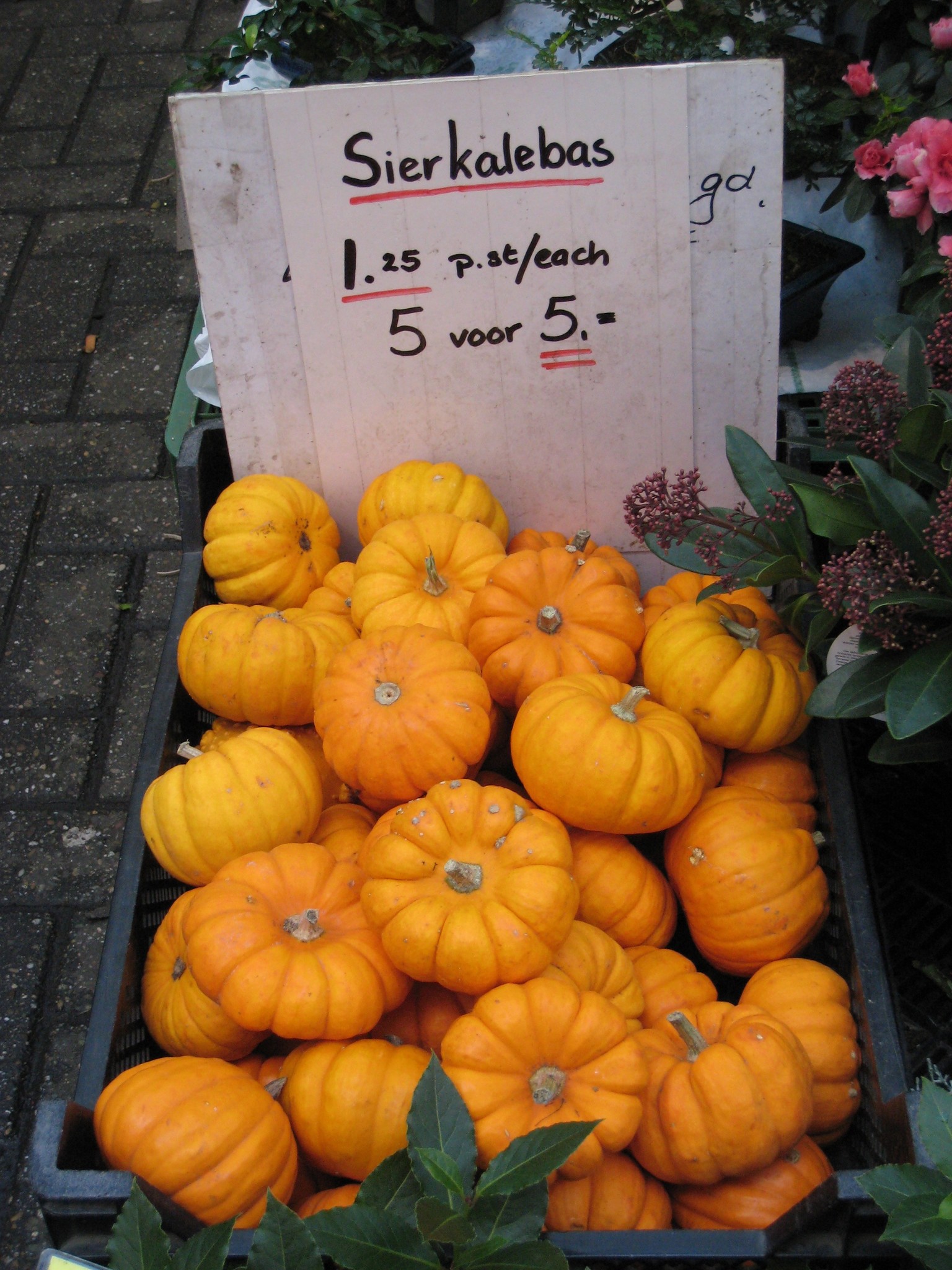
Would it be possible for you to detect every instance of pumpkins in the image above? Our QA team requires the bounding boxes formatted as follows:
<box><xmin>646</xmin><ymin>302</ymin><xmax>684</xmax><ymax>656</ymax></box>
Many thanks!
<box><xmin>90</xmin><ymin>458</ymin><xmax>866</xmax><ymax>1231</ymax></box>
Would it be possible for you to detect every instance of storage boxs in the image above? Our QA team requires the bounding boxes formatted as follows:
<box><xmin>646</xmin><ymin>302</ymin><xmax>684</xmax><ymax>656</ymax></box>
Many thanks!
<box><xmin>31</xmin><ymin>292</ymin><xmax>952</xmax><ymax>1270</ymax></box>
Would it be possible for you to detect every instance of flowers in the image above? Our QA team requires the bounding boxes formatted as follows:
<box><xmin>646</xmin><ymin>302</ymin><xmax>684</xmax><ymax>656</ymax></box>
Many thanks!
<box><xmin>534</xmin><ymin>0</ymin><xmax>952</xmax><ymax>768</ymax></box>
